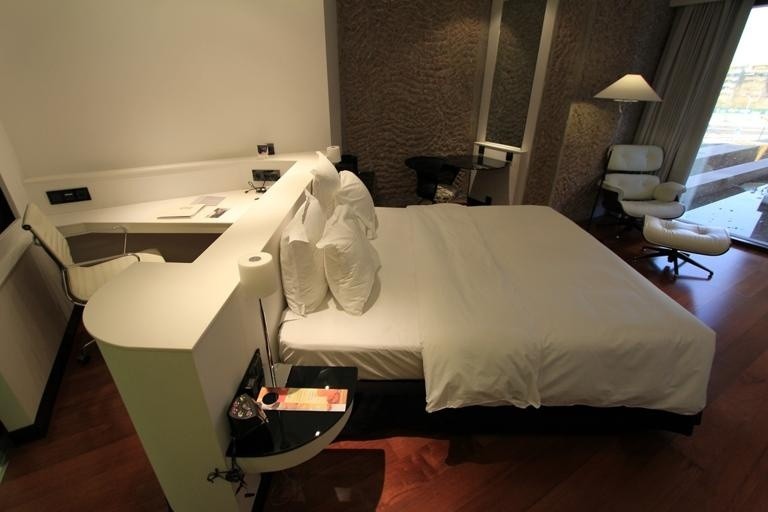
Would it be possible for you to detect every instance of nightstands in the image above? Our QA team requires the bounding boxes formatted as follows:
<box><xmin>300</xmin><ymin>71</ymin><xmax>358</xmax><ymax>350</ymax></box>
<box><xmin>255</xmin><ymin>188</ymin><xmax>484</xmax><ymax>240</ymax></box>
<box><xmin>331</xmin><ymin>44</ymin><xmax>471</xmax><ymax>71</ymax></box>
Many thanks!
<box><xmin>358</xmin><ymin>171</ymin><xmax>376</xmax><ymax>204</ymax></box>
<box><xmin>226</xmin><ymin>365</ymin><xmax>359</xmax><ymax>512</ymax></box>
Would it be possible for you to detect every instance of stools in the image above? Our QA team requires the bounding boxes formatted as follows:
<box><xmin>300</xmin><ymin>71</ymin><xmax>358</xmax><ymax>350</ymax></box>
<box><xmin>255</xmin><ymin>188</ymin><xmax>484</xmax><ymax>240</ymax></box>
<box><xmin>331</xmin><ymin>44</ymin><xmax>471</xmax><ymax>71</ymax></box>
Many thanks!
<box><xmin>634</xmin><ymin>210</ymin><xmax>731</xmax><ymax>280</ymax></box>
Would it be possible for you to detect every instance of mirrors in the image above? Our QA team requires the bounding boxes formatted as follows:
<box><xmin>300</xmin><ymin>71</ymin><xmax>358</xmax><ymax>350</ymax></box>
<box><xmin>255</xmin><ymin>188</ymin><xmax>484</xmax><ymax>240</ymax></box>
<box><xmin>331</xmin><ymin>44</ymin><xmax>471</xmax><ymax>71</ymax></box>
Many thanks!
<box><xmin>475</xmin><ymin>0</ymin><xmax>559</xmax><ymax>148</ymax></box>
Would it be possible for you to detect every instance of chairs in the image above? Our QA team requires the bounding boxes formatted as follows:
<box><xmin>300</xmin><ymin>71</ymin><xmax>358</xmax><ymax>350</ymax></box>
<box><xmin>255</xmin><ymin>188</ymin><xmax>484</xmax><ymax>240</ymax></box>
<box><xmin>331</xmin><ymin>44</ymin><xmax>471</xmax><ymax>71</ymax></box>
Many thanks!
<box><xmin>597</xmin><ymin>143</ymin><xmax>687</xmax><ymax>240</ymax></box>
<box><xmin>405</xmin><ymin>155</ymin><xmax>460</xmax><ymax>205</ymax></box>
<box><xmin>22</xmin><ymin>202</ymin><xmax>167</xmax><ymax>361</ymax></box>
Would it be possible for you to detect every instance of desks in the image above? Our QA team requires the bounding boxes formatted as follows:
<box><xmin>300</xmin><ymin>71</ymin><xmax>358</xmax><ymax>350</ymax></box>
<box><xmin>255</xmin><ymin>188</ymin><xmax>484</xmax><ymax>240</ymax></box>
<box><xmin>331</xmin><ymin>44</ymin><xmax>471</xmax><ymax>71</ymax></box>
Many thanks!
<box><xmin>46</xmin><ymin>185</ymin><xmax>272</xmax><ymax>238</ymax></box>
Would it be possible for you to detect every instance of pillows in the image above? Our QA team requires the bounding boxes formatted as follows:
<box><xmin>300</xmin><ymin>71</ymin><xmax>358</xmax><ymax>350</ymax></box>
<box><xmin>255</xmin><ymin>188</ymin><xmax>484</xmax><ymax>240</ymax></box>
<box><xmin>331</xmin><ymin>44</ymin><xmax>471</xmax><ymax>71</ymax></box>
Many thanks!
<box><xmin>279</xmin><ymin>151</ymin><xmax>381</xmax><ymax>317</ymax></box>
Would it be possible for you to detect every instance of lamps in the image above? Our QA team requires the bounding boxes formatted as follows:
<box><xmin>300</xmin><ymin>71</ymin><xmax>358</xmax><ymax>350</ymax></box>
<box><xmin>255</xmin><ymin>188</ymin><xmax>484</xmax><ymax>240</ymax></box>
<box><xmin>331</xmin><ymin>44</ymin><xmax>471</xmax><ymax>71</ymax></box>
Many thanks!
<box><xmin>236</xmin><ymin>251</ymin><xmax>280</xmax><ymax>387</ymax></box>
<box><xmin>327</xmin><ymin>145</ymin><xmax>342</xmax><ymax>167</ymax></box>
<box><xmin>583</xmin><ymin>72</ymin><xmax>663</xmax><ymax>231</ymax></box>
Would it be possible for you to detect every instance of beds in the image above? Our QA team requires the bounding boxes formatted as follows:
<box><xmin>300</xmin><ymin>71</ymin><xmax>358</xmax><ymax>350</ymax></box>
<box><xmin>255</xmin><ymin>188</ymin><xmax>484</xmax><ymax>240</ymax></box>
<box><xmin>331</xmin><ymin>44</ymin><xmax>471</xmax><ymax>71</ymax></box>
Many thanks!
<box><xmin>277</xmin><ymin>204</ymin><xmax>717</xmax><ymax>437</ymax></box>
